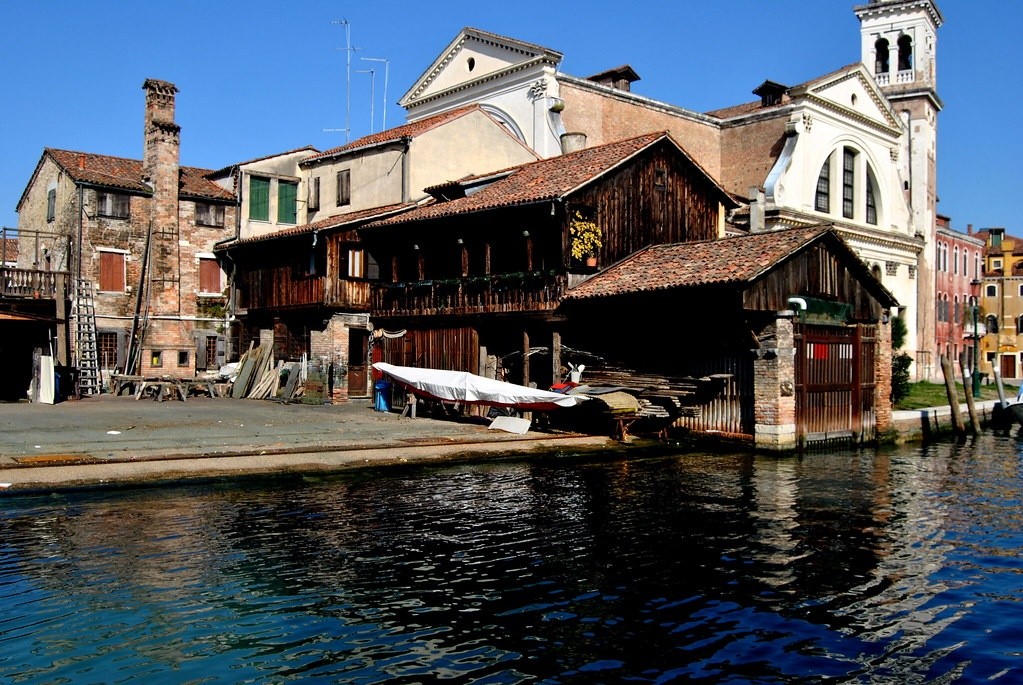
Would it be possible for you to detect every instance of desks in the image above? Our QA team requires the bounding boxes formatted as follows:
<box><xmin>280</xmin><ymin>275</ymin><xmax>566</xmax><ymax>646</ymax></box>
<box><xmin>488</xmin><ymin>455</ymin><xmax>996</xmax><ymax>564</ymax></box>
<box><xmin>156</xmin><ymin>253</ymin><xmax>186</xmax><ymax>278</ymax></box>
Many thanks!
<box><xmin>114</xmin><ymin>376</ymin><xmax>215</xmax><ymax>402</ymax></box>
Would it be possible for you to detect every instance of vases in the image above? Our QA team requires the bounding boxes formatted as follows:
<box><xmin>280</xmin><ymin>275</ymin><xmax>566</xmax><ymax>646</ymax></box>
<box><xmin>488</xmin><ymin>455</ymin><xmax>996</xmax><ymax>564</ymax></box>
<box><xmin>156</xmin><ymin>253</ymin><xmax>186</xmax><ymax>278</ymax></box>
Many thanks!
<box><xmin>587</xmin><ymin>258</ymin><xmax>597</xmax><ymax>266</ymax></box>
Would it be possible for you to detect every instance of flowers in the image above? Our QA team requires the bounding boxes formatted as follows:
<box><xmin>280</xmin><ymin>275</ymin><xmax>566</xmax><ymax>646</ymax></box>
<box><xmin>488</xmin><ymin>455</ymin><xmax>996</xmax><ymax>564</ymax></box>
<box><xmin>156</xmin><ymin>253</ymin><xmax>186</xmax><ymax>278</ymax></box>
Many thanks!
<box><xmin>570</xmin><ymin>210</ymin><xmax>603</xmax><ymax>261</ymax></box>
